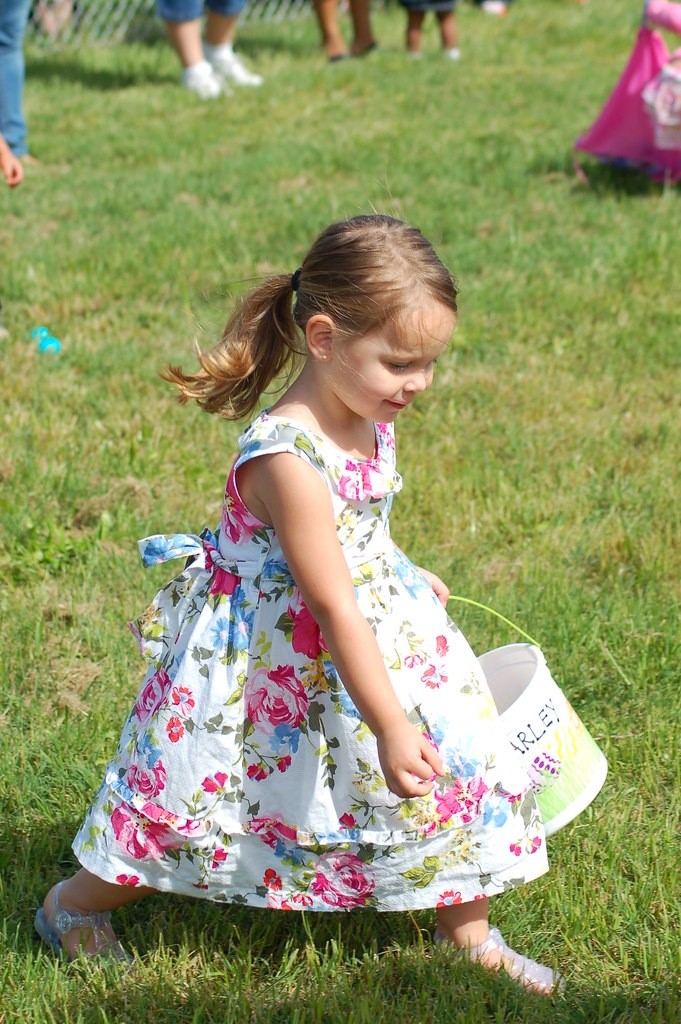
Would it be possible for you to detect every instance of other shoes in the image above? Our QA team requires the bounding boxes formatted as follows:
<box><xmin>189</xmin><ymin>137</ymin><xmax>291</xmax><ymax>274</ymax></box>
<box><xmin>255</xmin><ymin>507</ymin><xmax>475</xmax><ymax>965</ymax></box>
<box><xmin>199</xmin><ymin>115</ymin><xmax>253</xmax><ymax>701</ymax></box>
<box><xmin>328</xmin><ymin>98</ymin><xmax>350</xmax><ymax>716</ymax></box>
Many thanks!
<box><xmin>442</xmin><ymin>47</ymin><xmax>461</xmax><ymax>60</ymax></box>
<box><xmin>330</xmin><ymin>54</ymin><xmax>351</xmax><ymax>62</ymax></box>
<box><xmin>181</xmin><ymin>67</ymin><xmax>224</xmax><ymax>98</ymax></box>
<box><xmin>353</xmin><ymin>43</ymin><xmax>378</xmax><ymax>56</ymax></box>
<box><xmin>201</xmin><ymin>43</ymin><xmax>262</xmax><ymax>86</ymax></box>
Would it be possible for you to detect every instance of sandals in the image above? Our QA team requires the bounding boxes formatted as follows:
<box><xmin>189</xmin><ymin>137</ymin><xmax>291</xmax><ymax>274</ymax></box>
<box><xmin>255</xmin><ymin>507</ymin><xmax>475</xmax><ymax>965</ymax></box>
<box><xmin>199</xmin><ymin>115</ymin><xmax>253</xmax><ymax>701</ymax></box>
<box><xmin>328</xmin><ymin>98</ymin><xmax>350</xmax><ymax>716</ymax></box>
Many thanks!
<box><xmin>32</xmin><ymin>882</ymin><xmax>128</xmax><ymax>966</ymax></box>
<box><xmin>434</xmin><ymin>927</ymin><xmax>566</xmax><ymax>995</ymax></box>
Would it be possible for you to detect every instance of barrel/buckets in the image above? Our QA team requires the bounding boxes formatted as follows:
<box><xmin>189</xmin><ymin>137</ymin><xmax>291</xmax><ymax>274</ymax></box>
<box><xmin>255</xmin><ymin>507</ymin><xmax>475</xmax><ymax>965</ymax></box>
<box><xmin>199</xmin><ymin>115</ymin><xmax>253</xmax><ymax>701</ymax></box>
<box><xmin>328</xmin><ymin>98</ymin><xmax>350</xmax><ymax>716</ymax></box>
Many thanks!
<box><xmin>448</xmin><ymin>596</ymin><xmax>608</xmax><ymax>837</ymax></box>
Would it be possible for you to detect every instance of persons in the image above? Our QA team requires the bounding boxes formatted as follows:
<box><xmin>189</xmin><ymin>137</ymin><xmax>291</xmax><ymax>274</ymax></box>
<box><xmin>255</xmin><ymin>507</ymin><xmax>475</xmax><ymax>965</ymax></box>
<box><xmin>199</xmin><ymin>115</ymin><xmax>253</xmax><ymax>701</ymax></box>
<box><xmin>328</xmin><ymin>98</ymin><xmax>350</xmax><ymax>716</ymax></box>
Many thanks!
<box><xmin>35</xmin><ymin>214</ymin><xmax>565</xmax><ymax>994</ymax></box>
<box><xmin>400</xmin><ymin>0</ymin><xmax>459</xmax><ymax>60</ymax></box>
<box><xmin>0</xmin><ymin>0</ymin><xmax>38</xmax><ymax>188</ymax></box>
<box><xmin>314</xmin><ymin>0</ymin><xmax>377</xmax><ymax>63</ymax></box>
<box><xmin>642</xmin><ymin>0</ymin><xmax>681</xmax><ymax>75</ymax></box>
<box><xmin>157</xmin><ymin>0</ymin><xmax>263</xmax><ymax>100</ymax></box>
